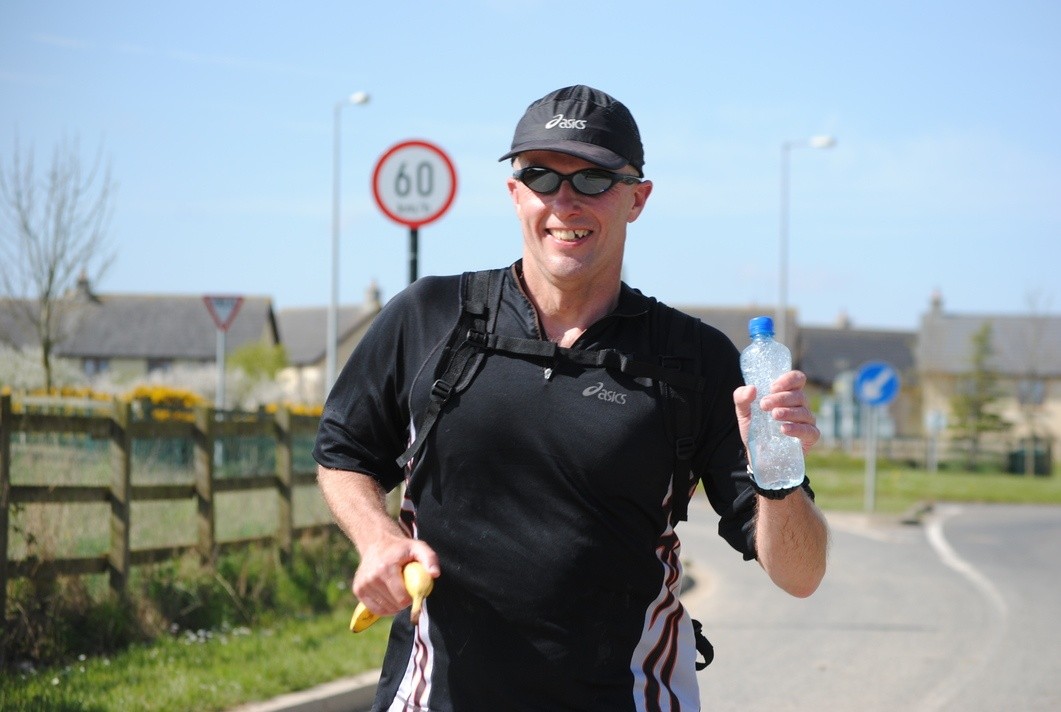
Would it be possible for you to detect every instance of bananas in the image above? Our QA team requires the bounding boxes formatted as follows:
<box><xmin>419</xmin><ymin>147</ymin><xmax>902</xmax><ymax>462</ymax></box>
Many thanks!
<box><xmin>350</xmin><ymin>561</ymin><xmax>433</xmax><ymax>633</ymax></box>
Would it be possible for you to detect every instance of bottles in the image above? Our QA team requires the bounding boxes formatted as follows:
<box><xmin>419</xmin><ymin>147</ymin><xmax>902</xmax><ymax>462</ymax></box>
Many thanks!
<box><xmin>740</xmin><ymin>316</ymin><xmax>806</xmax><ymax>489</ymax></box>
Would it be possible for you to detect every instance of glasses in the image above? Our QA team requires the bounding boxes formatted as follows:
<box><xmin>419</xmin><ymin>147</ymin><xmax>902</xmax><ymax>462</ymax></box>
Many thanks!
<box><xmin>513</xmin><ymin>166</ymin><xmax>644</xmax><ymax>196</ymax></box>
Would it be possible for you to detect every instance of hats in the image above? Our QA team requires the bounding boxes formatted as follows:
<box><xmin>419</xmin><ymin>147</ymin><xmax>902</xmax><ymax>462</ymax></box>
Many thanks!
<box><xmin>498</xmin><ymin>84</ymin><xmax>645</xmax><ymax>177</ymax></box>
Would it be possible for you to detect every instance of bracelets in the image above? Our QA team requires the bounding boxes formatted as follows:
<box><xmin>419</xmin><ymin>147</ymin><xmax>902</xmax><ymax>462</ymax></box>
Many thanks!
<box><xmin>747</xmin><ymin>464</ymin><xmax>797</xmax><ymax>500</ymax></box>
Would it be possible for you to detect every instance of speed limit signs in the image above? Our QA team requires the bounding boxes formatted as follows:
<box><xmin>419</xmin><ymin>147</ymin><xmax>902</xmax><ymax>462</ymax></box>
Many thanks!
<box><xmin>371</xmin><ymin>142</ymin><xmax>456</xmax><ymax>225</ymax></box>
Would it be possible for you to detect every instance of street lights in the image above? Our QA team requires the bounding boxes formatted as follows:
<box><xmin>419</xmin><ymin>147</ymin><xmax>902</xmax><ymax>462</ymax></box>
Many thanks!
<box><xmin>327</xmin><ymin>89</ymin><xmax>371</xmax><ymax>400</ymax></box>
<box><xmin>775</xmin><ymin>134</ymin><xmax>841</xmax><ymax>340</ymax></box>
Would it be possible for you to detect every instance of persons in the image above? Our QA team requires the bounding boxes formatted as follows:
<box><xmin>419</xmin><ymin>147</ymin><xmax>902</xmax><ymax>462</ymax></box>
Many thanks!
<box><xmin>311</xmin><ymin>84</ymin><xmax>826</xmax><ymax>712</ymax></box>
